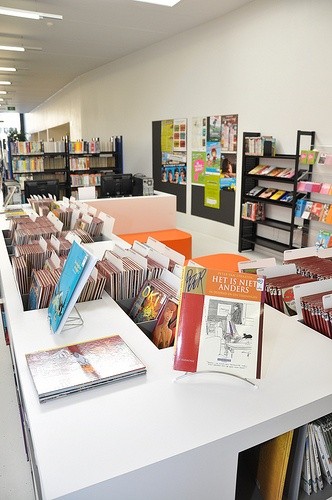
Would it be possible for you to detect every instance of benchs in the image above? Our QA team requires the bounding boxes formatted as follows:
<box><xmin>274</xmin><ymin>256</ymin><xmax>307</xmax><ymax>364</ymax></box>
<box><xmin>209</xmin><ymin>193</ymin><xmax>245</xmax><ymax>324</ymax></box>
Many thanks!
<box><xmin>116</xmin><ymin>229</ymin><xmax>191</xmax><ymax>260</ymax></box>
<box><xmin>183</xmin><ymin>254</ymin><xmax>251</xmax><ymax>274</ymax></box>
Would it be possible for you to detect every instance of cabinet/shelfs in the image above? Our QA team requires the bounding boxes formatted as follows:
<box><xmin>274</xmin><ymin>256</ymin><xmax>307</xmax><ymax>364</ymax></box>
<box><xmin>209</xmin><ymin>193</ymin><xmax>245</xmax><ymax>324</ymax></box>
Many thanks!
<box><xmin>0</xmin><ymin>111</ymin><xmax>332</xmax><ymax>500</ymax></box>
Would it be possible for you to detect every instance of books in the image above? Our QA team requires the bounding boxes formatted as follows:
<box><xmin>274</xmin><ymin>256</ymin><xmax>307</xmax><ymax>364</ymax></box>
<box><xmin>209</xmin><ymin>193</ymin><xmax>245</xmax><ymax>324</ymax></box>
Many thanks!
<box><xmin>47</xmin><ymin>240</ymin><xmax>91</xmax><ymax>334</ymax></box>
<box><xmin>244</xmin><ymin>136</ymin><xmax>276</xmax><ymax>157</ymax></box>
<box><xmin>245</xmin><ymin>186</ymin><xmax>264</xmax><ymax>196</ymax></box>
<box><xmin>280</xmin><ymin>191</ymin><xmax>300</xmax><ymax>202</ymax></box>
<box><xmin>295</xmin><ymin>199</ymin><xmax>332</xmax><ymax>224</ymax></box>
<box><xmin>314</xmin><ymin>230</ymin><xmax>332</xmax><ymax>249</ymax></box>
<box><xmin>241</xmin><ymin>255</ymin><xmax>332</xmax><ymax>339</ymax></box>
<box><xmin>8</xmin><ymin>193</ymin><xmax>185</xmax><ymax>349</ymax></box>
<box><xmin>296</xmin><ymin>180</ymin><xmax>332</xmax><ymax>195</ymax></box>
<box><xmin>301</xmin><ymin>414</ymin><xmax>332</xmax><ymax>495</ymax></box>
<box><xmin>25</xmin><ymin>334</ymin><xmax>147</xmax><ymax>404</ymax></box>
<box><xmin>269</xmin><ymin>190</ymin><xmax>286</xmax><ymax>200</ymax></box>
<box><xmin>172</xmin><ymin>266</ymin><xmax>266</xmax><ymax>380</ymax></box>
<box><xmin>258</xmin><ymin>188</ymin><xmax>276</xmax><ymax>198</ymax></box>
<box><xmin>241</xmin><ymin>202</ymin><xmax>264</xmax><ymax>221</ymax></box>
<box><xmin>10</xmin><ymin>141</ymin><xmax>66</xmax><ymax>190</ymax></box>
<box><xmin>69</xmin><ymin>141</ymin><xmax>114</xmax><ymax>199</ymax></box>
<box><xmin>248</xmin><ymin>163</ymin><xmax>301</xmax><ymax>178</ymax></box>
